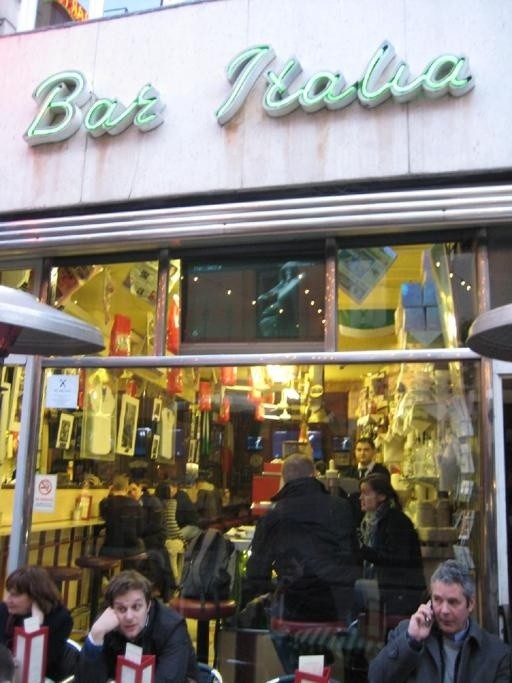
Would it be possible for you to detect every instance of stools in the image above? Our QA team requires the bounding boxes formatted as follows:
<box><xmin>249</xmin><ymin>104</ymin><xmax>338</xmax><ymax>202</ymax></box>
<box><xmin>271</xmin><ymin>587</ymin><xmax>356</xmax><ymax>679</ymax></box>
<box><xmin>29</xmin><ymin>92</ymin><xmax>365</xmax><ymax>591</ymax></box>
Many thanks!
<box><xmin>270</xmin><ymin>617</ymin><xmax>346</xmax><ymax>672</ymax></box>
<box><xmin>206</xmin><ymin>517</ymin><xmax>244</xmax><ymax>534</ymax></box>
<box><xmin>44</xmin><ymin>565</ymin><xmax>84</xmax><ymax>610</ymax></box>
<box><xmin>369</xmin><ymin>611</ymin><xmax>413</xmax><ymax>647</ymax></box>
<box><xmin>74</xmin><ymin>553</ymin><xmax>120</xmax><ymax>633</ymax></box>
<box><xmin>168</xmin><ymin>598</ymin><xmax>236</xmax><ymax>672</ymax></box>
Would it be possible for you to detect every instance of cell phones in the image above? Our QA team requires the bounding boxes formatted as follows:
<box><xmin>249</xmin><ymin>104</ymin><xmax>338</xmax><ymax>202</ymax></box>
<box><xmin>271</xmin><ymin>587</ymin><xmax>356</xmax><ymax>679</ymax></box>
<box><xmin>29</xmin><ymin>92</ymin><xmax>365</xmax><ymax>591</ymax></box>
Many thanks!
<box><xmin>425</xmin><ymin>600</ymin><xmax>434</xmax><ymax>626</ymax></box>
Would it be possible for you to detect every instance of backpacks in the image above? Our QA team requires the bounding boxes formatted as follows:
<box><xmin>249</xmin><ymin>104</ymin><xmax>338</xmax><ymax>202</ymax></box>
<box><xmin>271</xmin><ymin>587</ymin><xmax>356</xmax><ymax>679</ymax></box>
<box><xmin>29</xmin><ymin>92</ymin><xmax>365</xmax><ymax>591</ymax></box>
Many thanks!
<box><xmin>182</xmin><ymin>528</ymin><xmax>235</xmax><ymax>596</ymax></box>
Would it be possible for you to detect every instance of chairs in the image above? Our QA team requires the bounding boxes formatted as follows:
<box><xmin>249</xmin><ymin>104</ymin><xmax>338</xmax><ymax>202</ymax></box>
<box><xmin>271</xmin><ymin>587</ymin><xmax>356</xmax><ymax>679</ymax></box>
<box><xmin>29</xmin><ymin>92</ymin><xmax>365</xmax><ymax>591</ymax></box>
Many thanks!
<box><xmin>55</xmin><ymin>637</ymin><xmax>344</xmax><ymax>683</ymax></box>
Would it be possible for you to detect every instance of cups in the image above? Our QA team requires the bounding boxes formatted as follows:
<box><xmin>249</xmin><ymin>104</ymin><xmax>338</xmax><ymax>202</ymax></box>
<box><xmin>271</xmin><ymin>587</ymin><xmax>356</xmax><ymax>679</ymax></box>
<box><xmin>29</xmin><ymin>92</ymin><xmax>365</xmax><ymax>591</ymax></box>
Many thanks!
<box><xmin>72</xmin><ymin>506</ymin><xmax>82</xmax><ymax>520</ymax></box>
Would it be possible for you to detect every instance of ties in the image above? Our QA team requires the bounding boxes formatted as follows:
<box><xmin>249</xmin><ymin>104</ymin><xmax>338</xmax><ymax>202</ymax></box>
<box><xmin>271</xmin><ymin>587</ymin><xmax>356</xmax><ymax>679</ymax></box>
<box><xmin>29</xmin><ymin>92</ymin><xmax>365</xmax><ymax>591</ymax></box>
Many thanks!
<box><xmin>359</xmin><ymin>467</ymin><xmax>368</xmax><ymax>477</ymax></box>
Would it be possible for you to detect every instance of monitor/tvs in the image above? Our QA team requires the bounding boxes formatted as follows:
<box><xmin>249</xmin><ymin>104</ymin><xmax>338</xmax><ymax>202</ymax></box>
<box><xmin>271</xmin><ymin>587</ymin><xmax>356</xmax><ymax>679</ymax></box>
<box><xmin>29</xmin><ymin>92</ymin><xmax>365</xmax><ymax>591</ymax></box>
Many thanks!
<box><xmin>269</xmin><ymin>425</ymin><xmax>327</xmax><ymax>465</ymax></box>
<box><xmin>179</xmin><ymin>239</ymin><xmax>337</xmax><ymax>355</ymax></box>
<box><xmin>172</xmin><ymin>425</ymin><xmax>186</xmax><ymax>458</ymax></box>
<box><xmin>245</xmin><ymin>435</ymin><xmax>264</xmax><ymax>452</ymax></box>
<box><xmin>331</xmin><ymin>436</ymin><xmax>352</xmax><ymax>452</ymax></box>
<box><xmin>134</xmin><ymin>427</ymin><xmax>152</xmax><ymax>457</ymax></box>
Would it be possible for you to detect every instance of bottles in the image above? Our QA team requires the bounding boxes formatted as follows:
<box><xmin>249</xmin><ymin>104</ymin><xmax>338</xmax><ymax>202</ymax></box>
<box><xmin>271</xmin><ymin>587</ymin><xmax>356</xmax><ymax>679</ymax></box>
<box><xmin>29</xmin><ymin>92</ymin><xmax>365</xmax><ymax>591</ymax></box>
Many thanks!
<box><xmin>342</xmin><ymin>436</ymin><xmax>351</xmax><ymax>450</ymax></box>
<box><xmin>421</xmin><ymin>492</ymin><xmax>451</xmax><ymax>527</ymax></box>
<box><xmin>236</xmin><ymin>553</ymin><xmax>247</xmax><ymax>574</ymax></box>
<box><xmin>257</xmin><ymin>437</ymin><xmax>263</xmax><ymax>448</ymax></box>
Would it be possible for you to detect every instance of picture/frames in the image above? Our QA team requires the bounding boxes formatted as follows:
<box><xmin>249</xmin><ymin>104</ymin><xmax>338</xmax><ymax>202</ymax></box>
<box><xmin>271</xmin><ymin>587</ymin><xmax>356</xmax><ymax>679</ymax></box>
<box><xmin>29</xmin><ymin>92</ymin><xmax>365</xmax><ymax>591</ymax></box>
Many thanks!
<box><xmin>115</xmin><ymin>393</ymin><xmax>140</xmax><ymax>457</ymax></box>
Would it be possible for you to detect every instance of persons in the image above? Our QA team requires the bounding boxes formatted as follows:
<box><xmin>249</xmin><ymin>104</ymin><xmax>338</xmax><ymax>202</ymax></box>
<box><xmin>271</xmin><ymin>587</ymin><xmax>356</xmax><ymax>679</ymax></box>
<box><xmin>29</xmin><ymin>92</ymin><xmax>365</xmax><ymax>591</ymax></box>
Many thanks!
<box><xmin>366</xmin><ymin>559</ymin><xmax>512</xmax><ymax>682</ymax></box>
<box><xmin>256</xmin><ymin>260</ymin><xmax>303</xmax><ymax>338</ymax></box>
<box><xmin>0</xmin><ymin>437</ymin><xmax>428</xmax><ymax>683</ymax></box>
